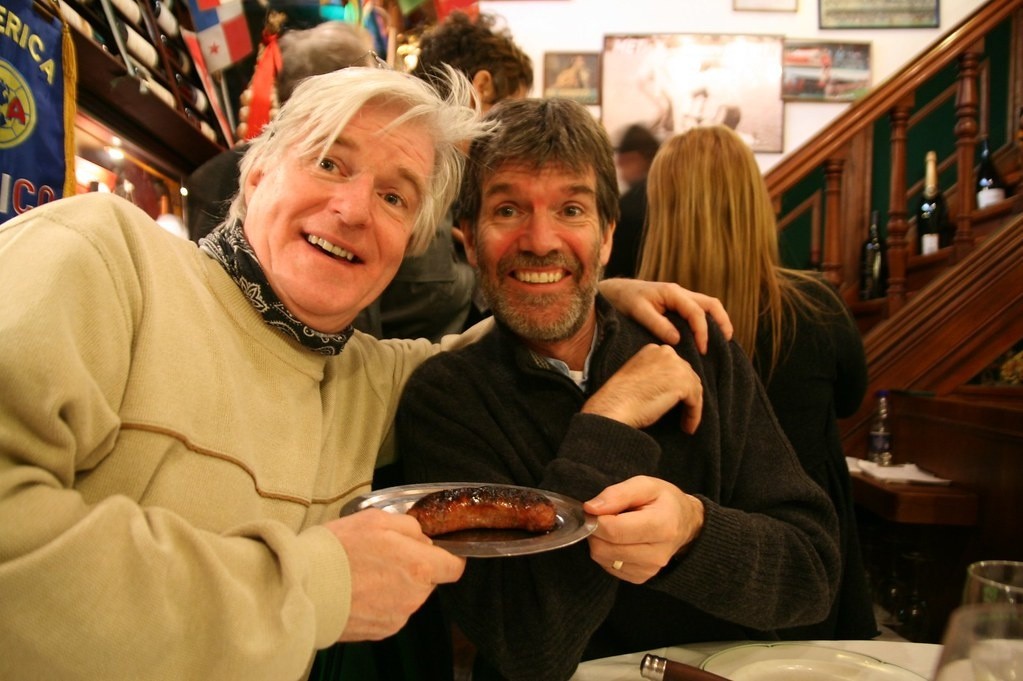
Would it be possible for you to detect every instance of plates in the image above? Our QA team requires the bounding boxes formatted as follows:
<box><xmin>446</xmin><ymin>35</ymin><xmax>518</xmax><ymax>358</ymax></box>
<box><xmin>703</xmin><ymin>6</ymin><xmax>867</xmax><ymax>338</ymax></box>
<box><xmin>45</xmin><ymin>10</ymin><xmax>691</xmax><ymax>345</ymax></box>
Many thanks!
<box><xmin>340</xmin><ymin>482</ymin><xmax>598</xmax><ymax>558</ymax></box>
<box><xmin>697</xmin><ymin>644</ymin><xmax>925</xmax><ymax>681</ymax></box>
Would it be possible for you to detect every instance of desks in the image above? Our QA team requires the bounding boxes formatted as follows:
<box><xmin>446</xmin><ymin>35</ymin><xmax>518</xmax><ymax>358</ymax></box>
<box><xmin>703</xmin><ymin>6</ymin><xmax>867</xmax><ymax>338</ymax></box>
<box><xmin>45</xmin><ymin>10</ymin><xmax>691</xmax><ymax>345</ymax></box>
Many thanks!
<box><xmin>850</xmin><ymin>473</ymin><xmax>985</xmax><ymax>634</ymax></box>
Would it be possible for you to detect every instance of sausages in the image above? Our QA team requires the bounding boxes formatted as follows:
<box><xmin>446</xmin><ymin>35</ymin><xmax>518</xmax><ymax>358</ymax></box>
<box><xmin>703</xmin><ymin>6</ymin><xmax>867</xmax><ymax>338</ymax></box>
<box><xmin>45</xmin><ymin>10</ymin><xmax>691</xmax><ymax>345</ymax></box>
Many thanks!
<box><xmin>405</xmin><ymin>485</ymin><xmax>556</xmax><ymax>537</ymax></box>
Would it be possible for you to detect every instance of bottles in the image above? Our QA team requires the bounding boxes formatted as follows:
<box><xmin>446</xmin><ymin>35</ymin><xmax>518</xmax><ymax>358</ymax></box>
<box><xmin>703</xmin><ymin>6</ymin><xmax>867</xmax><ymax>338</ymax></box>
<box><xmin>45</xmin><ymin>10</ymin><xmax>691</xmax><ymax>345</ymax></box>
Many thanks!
<box><xmin>915</xmin><ymin>151</ymin><xmax>950</xmax><ymax>255</ymax></box>
<box><xmin>52</xmin><ymin>0</ymin><xmax>219</xmax><ymax>144</ymax></box>
<box><xmin>857</xmin><ymin>211</ymin><xmax>887</xmax><ymax>300</ymax></box>
<box><xmin>867</xmin><ymin>391</ymin><xmax>892</xmax><ymax>465</ymax></box>
<box><xmin>975</xmin><ymin>138</ymin><xmax>1007</xmax><ymax>209</ymax></box>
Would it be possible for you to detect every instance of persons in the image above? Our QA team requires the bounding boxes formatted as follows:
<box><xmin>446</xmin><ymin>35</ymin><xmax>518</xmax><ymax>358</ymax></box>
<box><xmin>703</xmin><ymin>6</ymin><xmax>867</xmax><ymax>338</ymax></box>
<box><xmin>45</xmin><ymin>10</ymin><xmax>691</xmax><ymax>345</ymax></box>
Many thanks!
<box><xmin>0</xmin><ymin>61</ymin><xmax>734</xmax><ymax>681</ymax></box>
<box><xmin>631</xmin><ymin>124</ymin><xmax>882</xmax><ymax>640</ymax></box>
<box><xmin>186</xmin><ymin>10</ymin><xmax>663</xmax><ymax>344</ymax></box>
<box><xmin>381</xmin><ymin>96</ymin><xmax>845</xmax><ymax>681</ymax></box>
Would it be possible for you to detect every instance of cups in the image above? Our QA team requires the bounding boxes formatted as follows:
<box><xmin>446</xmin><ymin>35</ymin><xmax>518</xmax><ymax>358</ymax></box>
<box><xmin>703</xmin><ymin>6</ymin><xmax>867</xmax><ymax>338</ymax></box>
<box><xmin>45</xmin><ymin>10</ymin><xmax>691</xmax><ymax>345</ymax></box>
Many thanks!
<box><xmin>934</xmin><ymin>605</ymin><xmax>1023</xmax><ymax>680</ymax></box>
<box><xmin>962</xmin><ymin>560</ymin><xmax>1023</xmax><ymax>604</ymax></box>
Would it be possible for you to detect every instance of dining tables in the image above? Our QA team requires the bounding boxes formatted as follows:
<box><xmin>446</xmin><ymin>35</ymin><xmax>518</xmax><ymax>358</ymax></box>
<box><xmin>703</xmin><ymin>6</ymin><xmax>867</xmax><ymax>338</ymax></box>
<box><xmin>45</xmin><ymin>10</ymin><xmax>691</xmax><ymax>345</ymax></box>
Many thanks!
<box><xmin>570</xmin><ymin>639</ymin><xmax>947</xmax><ymax>680</ymax></box>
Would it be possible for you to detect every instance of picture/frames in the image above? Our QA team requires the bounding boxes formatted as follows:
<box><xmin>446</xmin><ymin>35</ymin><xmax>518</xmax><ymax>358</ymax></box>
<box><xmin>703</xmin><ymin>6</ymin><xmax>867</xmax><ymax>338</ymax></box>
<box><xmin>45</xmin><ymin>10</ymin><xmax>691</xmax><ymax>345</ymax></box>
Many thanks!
<box><xmin>601</xmin><ymin>33</ymin><xmax>785</xmax><ymax>152</ymax></box>
<box><xmin>818</xmin><ymin>0</ymin><xmax>940</xmax><ymax>29</ymax></box>
<box><xmin>544</xmin><ymin>51</ymin><xmax>601</xmax><ymax>107</ymax></box>
<box><xmin>781</xmin><ymin>39</ymin><xmax>875</xmax><ymax>103</ymax></box>
<box><xmin>733</xmin><ymin>0</ymin><xmax>799</xmax><ymax>12</ymax></box>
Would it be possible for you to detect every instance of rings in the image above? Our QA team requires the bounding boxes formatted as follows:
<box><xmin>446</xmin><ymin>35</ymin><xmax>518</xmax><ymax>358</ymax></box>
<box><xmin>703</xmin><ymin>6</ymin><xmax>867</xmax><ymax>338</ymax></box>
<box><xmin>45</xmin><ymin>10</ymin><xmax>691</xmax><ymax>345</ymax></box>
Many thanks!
<box><xmin>612</xmin><ymin>560</ymin><xmax>623</xmax><ymax>570</ymax></box>
